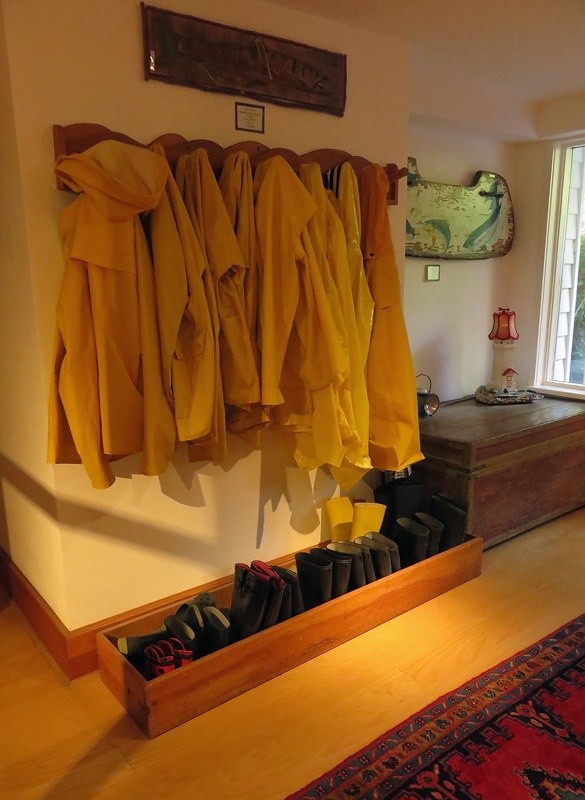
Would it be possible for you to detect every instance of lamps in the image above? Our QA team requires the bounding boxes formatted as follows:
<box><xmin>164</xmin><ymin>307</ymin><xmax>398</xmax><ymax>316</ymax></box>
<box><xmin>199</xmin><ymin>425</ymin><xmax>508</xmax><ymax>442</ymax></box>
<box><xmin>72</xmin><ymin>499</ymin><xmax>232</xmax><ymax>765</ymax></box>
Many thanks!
<box><xmin>488</xmin><ymin>307</ymin><xmax>521</xmax><ymax>389</ymax></box>
<box><xmin>416</xmin><ymin>374</ymin><xmax>439</xmax><ymax>417</ymax></box>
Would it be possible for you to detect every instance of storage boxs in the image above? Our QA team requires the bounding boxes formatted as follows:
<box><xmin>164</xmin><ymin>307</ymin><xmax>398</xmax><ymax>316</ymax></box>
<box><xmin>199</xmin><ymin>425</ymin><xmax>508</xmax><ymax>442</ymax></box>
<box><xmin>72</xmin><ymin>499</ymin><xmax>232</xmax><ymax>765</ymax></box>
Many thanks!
<box><xmin>97</xmin><ymin>531</ymin><xmax>483</xmax><ymax>741</ymax></box>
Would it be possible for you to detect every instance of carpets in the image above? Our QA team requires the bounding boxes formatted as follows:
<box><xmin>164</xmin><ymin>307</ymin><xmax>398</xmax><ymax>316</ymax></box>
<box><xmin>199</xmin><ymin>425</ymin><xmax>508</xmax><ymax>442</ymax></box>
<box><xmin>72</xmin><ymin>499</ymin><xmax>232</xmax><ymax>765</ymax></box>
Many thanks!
<box><xmin>285</xmin><ymin>615</ymin><xmax>585</xmax><ymax>800</ymax></box>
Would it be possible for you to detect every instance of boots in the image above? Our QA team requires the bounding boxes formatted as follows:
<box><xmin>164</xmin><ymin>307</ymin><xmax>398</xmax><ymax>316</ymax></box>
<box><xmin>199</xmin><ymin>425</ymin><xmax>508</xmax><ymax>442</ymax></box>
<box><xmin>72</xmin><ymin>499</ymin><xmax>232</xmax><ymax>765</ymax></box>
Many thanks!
<box><xmin>166</xmin><ymin>615</ymin><xmax>196</xmax><ymax>651</ymax></box>
<box><xmin>273</xmin><ymin>566</ymin><xmax>292</xmax><ymax>623</ymax></box>
<box><xmin>366</xmin><ymin>532</ymin><xmax>401</xmax><ymax>572</ymax></box>
<box><xmin>431</xmin><ymin>492</ymin><xmax>469</xmax><ymax>553</ymax></box>
<box><xmin>221</xmin><ymin>563</ymin><xmax>270</xmax><ymax>636</ymax></box>
<box><xmin>201</xmin><ymin>606</ymin><xmax>230</xmax><ymax>657</ymax></box>
<box><xmin>415</xmin><ymin>511</ymin><xmax>443</xmax><ymax>558</ymax></box>
<box><xmin>324</xmin><ymin>497</ymin><xmax>354</xmax><ymax>543</ymax></box>
<box><xmin>160</xmin><ymin>637</ymin><xmax>193</xmax><ymax>668</ymax></box>
<box><xmin>145</xmin><ymin>644</ymin><xmax>175</xmax><ymax>677</ymax></box>
<box><xmin>310</xmin><ymin>548</ymin><xmax>352</xmax><ymax>597</ymax></box>
<box><xmin>354</xmin><ymin>536</ymin><xmax>392</xmax><ymax>578</ymax></box>
<box><xmin>117</xmin><ymin>629</ymin><xmax>168</xmax><ymax>658</ymax></box>
<box><xmin>252</xmin><ymin>560</ymin><xmax>286</xmax><ymax>630</ymax></box>
<box><xmin>296</xmin><ymin>552</ymin><xmax>333</xmax><ymax>611</ymax></box>
<box><xmin>327</xmin><ymin>542</ymin><xmax>365</xmax><ymax>589</ymax></box>
<box><xmin>339</xmin><ymin>540</ymin><xmax>376</xmax><ymax>583</ymax></box>
<box><xmin>183</xmin><ymin>604</ymin><xmax>204</xmax><ymax>658</ymax></box>
<box><xmin>274</xmin><ymin>565</ymin><xmax>303</xmax><ymax>616</ymax></box>
<box><xmin>395</xmin><ymin>518</ymin><xmax>431</xmax><ymax>565</ymax></box>
<box><xmin>350</xmin><ymin>503</ymin><xmax>386</xmax><ymax>542</ymax></box>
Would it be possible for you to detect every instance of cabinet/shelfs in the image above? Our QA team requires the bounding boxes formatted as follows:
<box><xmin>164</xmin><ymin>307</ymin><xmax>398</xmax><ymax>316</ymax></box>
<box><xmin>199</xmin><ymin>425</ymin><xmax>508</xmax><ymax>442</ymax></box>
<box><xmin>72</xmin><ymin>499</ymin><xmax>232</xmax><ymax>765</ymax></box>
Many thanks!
<box><xmin>408</xmin><ymin>391</ymin><xmax>585</xmax><ymax>549</ymax></box>
